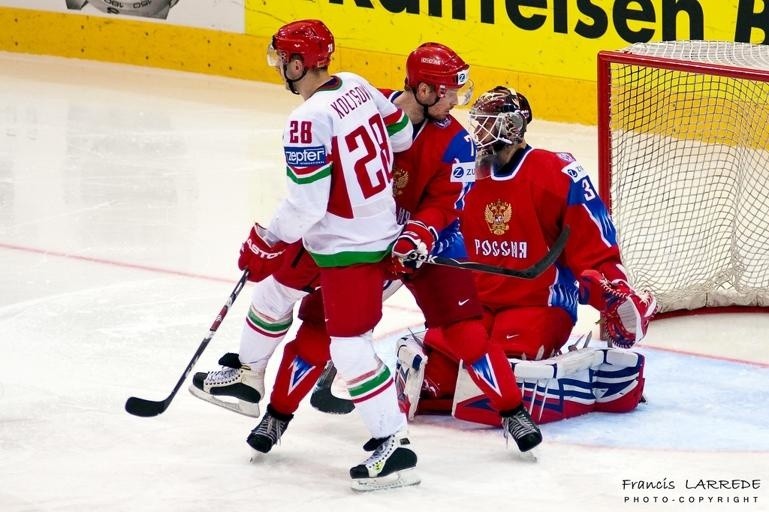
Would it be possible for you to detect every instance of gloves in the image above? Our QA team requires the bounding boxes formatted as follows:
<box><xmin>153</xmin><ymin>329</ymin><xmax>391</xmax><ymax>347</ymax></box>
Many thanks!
<box><xmin>392</xmin><ymin>218</ymin><xmax>438</xmax><ymax>275</ymax></box>
<box><xmin>605</xmin><ymin>283</ymin><xmax>654</xmax><ymax>348</ymax></box>
<box><xmin>238</xmin><ymin>223</ymin><xmax>288</xmax><ymax>282</ymax></box>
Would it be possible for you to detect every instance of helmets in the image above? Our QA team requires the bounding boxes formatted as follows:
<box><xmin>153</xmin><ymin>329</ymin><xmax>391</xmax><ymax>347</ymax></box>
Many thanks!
<box><xmin>468</xmin><ymin>86</ymin><xmax>533</xmax><ymax>149</ymax></box>
<box><xmin>404</xmin><ymin>43</ymin><xmax>474</xmax><ymax>105</ymax></box>
<box><xmin>266</xmin><ymin>20</ymin><xmax>335</xmax><ymax>68</ymax></box>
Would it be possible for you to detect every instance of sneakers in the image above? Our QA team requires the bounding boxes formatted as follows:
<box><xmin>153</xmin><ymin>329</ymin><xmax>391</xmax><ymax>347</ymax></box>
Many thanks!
<box><xmin>350</xmin><ymin>431</ymin><xmax>417</xmax><ymax>479</ymax></box>
<box><xmin>193</xmin><ymin>352</ymin><xmax>265</xmax><ymax>402</ymax></box>
<box><xmin>246</xmin><ymin>405</ymin><xmax>294</xmax><ymax>454</ymax></box>
<box><xmin>501</xmin><ymin>404</ymin><xmax>541</xmax><ymax>453</ymax></box>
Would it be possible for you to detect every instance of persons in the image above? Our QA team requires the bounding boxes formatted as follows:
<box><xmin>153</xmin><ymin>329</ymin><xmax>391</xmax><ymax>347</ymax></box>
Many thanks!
<box><xmin>193</xmin><ymin>18</ymin><xmax>418</xmax><ymax>478</ymax></box>
<box><xmin>413</xmin><ymin>85</ymin><xmax>660</xmax><ymax>415</ymax></box>
<box><xmin>245</xmin><ymin>41</ymin><xmax>543</xmax><ymax>453</ymax></box>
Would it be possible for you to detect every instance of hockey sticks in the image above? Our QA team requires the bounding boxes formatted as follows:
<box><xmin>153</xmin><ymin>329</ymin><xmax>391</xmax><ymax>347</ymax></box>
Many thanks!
<box><xmin>310</xmin><ymin>362</ymin><xmax>355</xmax><ymax>414</ymax></box>
<box><xmin>420</xmin><ymin>224</ymin><xmax>570</xmax><ymax>281</ymax></box>
<box><xmin>126</xmin><ymin>272</ymin><xmax>247</xmax><ymax>418</ymax></box>
